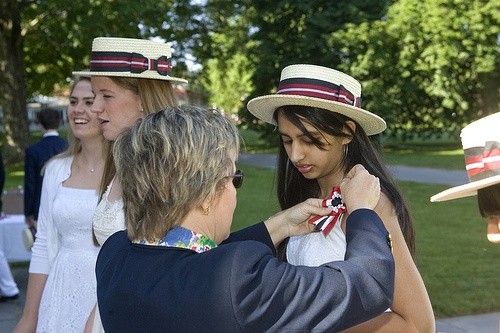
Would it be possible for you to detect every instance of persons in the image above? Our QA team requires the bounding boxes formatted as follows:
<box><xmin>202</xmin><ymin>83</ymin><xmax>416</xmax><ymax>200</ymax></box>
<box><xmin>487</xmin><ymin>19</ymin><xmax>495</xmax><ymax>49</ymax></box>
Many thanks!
<box><xmin>24</xmin><ymin>107</ymin><xmax>70</xmax><ymax>242</ymax></box>
<box><xmin>245</xmin><ymin>63</ymin><xmax>437</xmax><ymax>333</ymax></box>
<box><xmin>0</xmin><ymin>153</ymin><xmax>20</xmax><ymax>302</ymax></box>
<box><xmin>10</xmin><ymin>68</ymin><xmax>109</xmax><ymax>333</ymax></box>
<box><xmin>429</xmin><ymin>111</ymin><xmax>500</xmax><ymax>246</ymax></box>
<box><xmin>95</xmin><ymin>105</ymin><xmax>396</xmax><ymax>332</ymax></box>
<box><xmin>84</xmin><ymin>37</ymin><xmax>188</xmax><ymax>333</ymax></box>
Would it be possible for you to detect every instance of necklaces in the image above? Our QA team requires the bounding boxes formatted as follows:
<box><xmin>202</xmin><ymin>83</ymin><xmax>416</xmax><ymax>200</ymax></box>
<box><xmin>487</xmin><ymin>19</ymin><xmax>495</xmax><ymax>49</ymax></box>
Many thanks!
<box><xmin>81</xmin><ymin>151</ymin><xmax>104</xmax><ymax>173</ymax></box>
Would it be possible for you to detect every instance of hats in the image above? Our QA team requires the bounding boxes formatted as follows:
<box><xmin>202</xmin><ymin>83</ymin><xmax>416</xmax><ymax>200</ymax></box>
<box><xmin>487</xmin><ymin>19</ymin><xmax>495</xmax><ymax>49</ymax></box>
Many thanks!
<box><xmin>428</xmin><ymin>110</ymin><xmax>500</xmax><ymax>203</ymax></box>
<box><xmin>72</xmin><ymin>37</ymin><xmax>189</xmax><ymax>85</ymax></box>
<box><xmin>247</xmin><ymin>64</ymin><xmax>387</xmax><ymax>136</ymax></box>
